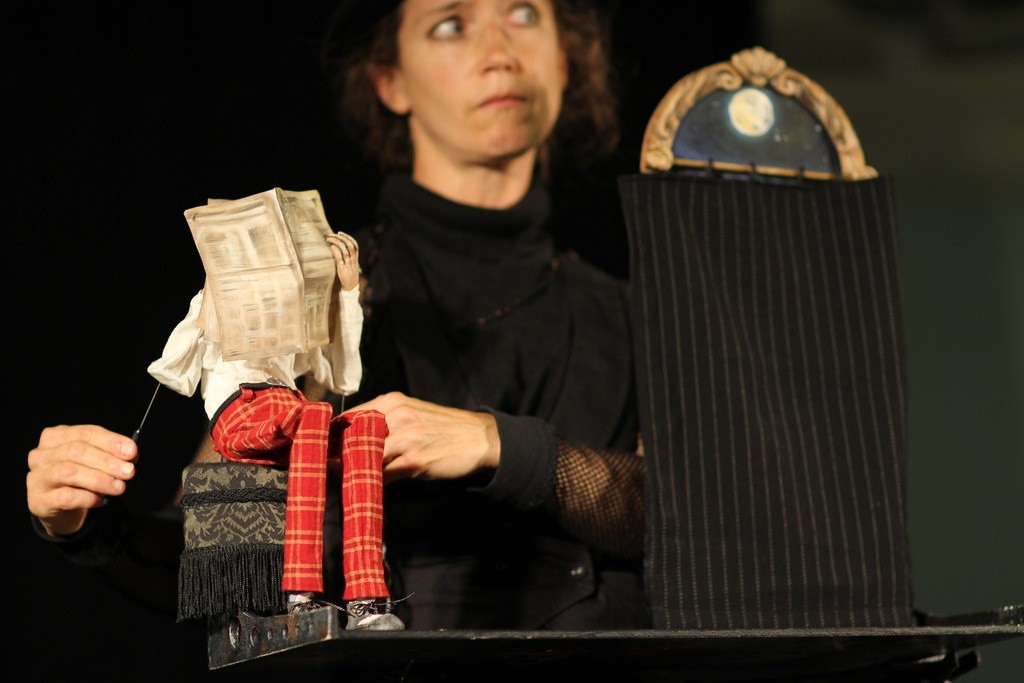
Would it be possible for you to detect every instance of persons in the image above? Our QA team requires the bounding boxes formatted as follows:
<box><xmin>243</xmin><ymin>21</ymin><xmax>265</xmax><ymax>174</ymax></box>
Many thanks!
<box><xmin>24</xmin><ymin>1</ymin><xmax>654</xmax><ymax>633</ymax></box>
<box><xmin>147</xmin><ymin>231</ymin><xmax>406</xmax><ymax>632</ymax></box>
